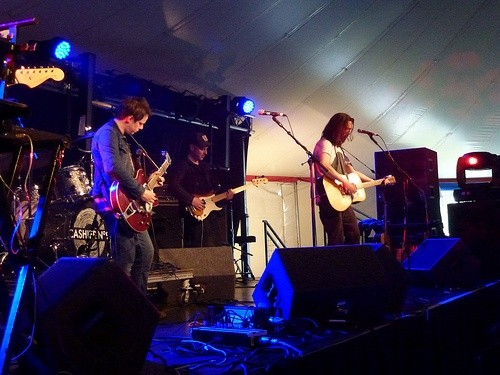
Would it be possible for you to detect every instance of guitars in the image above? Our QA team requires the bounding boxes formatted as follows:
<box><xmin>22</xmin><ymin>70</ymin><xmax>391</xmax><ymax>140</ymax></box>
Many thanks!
<box><xmin>187</xmin><ymin>174</ymin><xmax>269</xmax><ymax>219</ymax></box>
<box><xmin>7</xmin><ymin>65</ymin><xmax>65</xmax><ymax>89</ymax></box>
<box><xmin>110</xmin><ymin>150</ymin><xmax>172</xmax><ymax>232</ymax></box>
<box><xmin>316</xmin><ymin>171</ymin><xmax>396</xmax><ymax>212</ymax></box>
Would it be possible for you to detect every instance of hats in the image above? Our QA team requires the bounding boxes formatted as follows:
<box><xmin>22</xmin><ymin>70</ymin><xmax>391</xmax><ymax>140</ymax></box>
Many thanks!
<box><xmin>188</xmin><ymin>133</ymin><xmax>213</xmax><ymax>147</ymax></box>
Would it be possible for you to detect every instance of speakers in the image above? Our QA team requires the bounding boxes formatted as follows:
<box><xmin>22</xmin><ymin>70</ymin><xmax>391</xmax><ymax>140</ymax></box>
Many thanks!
<box><xmin>448</xmin><ymin>203</ymin><xmax>500</xmax><ymax>282</ymax></box>
<box><xmin>15</xmin><ymin>256</ymin><xmax>158</xmax><ymax>375</ymax></box>
<box><xmin>375</xmin><ymin>147</ymin><xmax>441</xmax><ymax>244</ymax></box>
<box><xmin>403</xmin><ymin>237</ymin><xmax>467</xmax><ymax>292</ymax></box>
<box><xmin>252</xmin><ymin>242</ymin><xmax>409</xmax><ymax>334</ymax></box>
<box><xmin>158</xmin><ymin>247</ymin><xmax>236</xmax><ymax>305</ymax></box>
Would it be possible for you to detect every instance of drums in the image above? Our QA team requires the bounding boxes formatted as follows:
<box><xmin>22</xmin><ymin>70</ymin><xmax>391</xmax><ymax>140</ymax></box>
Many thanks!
<box><xmin>50</xmin><ymin>164</ymin><xmax>92</xmax><ymax>198</ymax></box>
<box><xmin>25</xmin><ymin>202</ymin><xmax>109</xmax><ymax>258</ymax></box>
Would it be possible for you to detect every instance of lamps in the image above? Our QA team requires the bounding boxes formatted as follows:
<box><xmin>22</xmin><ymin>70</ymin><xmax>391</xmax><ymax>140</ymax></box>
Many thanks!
<box><xmin>229</xmin><ymin>94</ymin><xmax>255</xmax><ymax>117</ymax></box>
<box><xmin>37</xmin><ymin>39</ymin><xmax>72</xmax><ymax>61</ymax></box>
<box><xmin>453</xmin><ymin>152</ymin><xmax>500</xmax><ymax>201</ymax></box>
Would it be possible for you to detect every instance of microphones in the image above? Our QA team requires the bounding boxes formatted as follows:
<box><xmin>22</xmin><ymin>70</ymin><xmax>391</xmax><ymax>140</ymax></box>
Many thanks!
<box><xmin>357</xmin><ymin>129</ymin><xmax>375</xmax><ymax>136</ymax></box>
<box><xmin>258</xmin><ymin>109</ymin><xmax>286</xmax><ymax>117</ymax></box>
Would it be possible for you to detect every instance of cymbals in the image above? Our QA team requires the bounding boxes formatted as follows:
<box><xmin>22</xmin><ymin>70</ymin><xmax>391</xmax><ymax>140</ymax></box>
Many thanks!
<box><xmin>0</xmin><ymin>125</ymin><xmax>63</xmax><ymax>141</ymax></box>
<box><xmin>0</xmin><ymin>99</ymin><xmax>32</xmax><ymax>117</ymax></box>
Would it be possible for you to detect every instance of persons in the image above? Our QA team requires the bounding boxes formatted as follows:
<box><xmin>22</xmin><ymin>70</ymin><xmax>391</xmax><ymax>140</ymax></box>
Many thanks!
<box><xmin>92</xmin><ymin>96</ymin><xmax>166</xmax><ymax>318</ymax></box>
<box><xmin>168</xmin><ymin>130</ymin><xmax>235</xmax><ymax>246</ymax></box>
<box><xmin>313</xmin><ymin>112</ymin><xmax>393</xmax><ymax>247</ymax></box>
